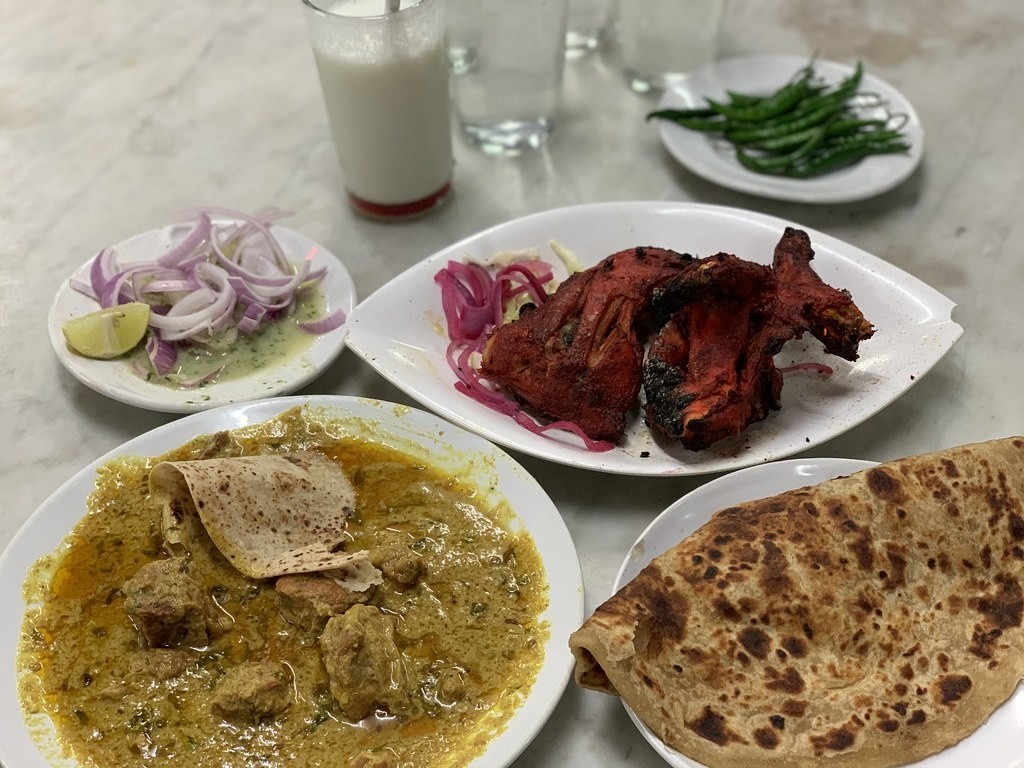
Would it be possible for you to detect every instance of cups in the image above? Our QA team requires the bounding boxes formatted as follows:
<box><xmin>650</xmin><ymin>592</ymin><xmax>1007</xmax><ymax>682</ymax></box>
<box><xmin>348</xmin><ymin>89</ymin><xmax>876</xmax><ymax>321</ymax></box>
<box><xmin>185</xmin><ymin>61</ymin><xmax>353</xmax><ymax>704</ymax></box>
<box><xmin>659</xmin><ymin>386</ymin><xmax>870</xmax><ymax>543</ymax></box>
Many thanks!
<box><xmin>599</xmin><ymin>0</ymin><xmax>729</xmax><ymax>98</ymax></box>
<box><xmin>565</xmin><ymin>0</ymin><xmax>618</xmax><ymax>65</ymax></box>
<box><xmin>448</xmin><ymin>1</ymin><xmax>567</xmax><ymax>160</ymax></box>
<box><xmin>303</xmin><ymin>0</ymin><xmax>453</xmax><ymax>224</ymax></box>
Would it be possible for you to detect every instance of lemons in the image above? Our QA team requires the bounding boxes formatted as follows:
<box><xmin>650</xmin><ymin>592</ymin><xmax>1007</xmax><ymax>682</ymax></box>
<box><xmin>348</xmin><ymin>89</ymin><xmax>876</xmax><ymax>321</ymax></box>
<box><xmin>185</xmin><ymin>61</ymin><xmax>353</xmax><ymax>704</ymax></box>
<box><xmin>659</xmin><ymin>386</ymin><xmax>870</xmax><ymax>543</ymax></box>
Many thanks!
<box><xmin>61</xmin><ymin>301</ymin><xmax>151</xmax><ymax>360</ymax></box>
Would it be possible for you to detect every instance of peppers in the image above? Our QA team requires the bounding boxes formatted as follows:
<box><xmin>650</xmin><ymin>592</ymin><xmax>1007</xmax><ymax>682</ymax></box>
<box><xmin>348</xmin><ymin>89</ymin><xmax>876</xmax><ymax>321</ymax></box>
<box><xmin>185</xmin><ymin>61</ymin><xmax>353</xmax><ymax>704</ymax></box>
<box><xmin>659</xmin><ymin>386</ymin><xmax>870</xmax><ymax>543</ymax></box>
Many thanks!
<box><xmin>645</xmin><ymin>51</ymin><xmax>911</xmax><ymax>177</ymax></box>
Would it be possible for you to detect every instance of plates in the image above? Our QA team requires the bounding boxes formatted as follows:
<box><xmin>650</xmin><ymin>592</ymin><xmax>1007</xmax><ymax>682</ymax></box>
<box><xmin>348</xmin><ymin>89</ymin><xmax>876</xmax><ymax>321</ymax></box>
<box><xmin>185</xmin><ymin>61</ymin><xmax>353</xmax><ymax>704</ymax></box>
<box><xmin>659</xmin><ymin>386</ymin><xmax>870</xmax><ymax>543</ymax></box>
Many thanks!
<box><xmin>651</xmin><ymin>53</ymin><xmax>923</xmax><ymax>204</ymax></box>
<box><xmin>342</xmin><ymin>202</ymin><xmax>964</xmax><ymax>478</ymax></box>
<box><xmin>611</xmin><ymin>458</ymin><xmax>1024</xmax><ymax>768</ymax></box>
<box><xmin>0</xmin><ymin>395</ymin><xmax>585</xmax><ymax>768</ymax></box>
<box><xmin>46</xmin><ymin>220</ymin><xmax>351</xmax><ymax>414</ymax></box>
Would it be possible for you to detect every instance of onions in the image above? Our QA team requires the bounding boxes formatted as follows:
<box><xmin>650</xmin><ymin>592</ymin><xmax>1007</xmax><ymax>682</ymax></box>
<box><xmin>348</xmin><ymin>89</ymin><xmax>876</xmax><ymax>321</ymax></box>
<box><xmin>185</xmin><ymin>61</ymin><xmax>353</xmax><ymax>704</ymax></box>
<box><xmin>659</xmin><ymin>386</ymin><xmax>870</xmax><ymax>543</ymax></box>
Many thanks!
<box><xmin>69</xmin><ymin>200</ymin><xmax>348</xmax><ymax>387</ymax></box>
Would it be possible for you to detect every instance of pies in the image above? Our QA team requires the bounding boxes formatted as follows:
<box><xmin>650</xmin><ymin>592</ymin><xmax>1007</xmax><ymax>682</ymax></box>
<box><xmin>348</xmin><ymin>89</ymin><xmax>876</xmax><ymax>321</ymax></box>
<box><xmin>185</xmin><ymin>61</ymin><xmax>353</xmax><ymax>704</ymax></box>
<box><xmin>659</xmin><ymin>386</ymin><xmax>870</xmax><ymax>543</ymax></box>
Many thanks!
<box><xmin>146</xmin><ymin>449</ymin><xmax>385</xmax><ymax>594</ymax></box>
<box><xmin>568</xmin><ymin>436</ymin><xmax>1024</xmax><ymax>768</ymax></box>
